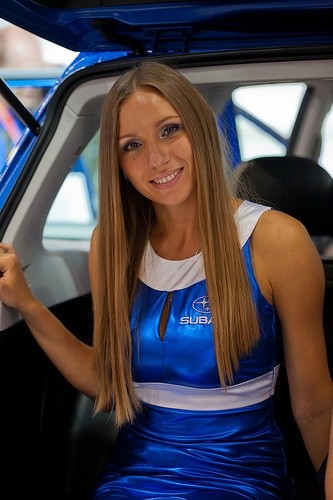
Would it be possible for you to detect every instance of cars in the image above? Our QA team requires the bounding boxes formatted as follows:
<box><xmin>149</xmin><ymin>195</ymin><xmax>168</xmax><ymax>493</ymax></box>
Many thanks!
<box><xmin>0</xmin><ymin>0</ymin><xmax>333</xmax><ymax>500</ymax></box>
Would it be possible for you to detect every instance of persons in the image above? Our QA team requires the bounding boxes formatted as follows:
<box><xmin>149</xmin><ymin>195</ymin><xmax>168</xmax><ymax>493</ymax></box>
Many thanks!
<box><xmin>0</xmin><ymin>59</ymin><xmax>333</xmax><ymax>500</ymax></box>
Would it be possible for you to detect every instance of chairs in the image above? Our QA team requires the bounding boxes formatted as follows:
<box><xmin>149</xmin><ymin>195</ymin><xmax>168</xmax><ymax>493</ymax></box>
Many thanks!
<box><xmin>234</xmin><ymin>156</ymin><xmax>333</xmax><ymax>379</ymax></box>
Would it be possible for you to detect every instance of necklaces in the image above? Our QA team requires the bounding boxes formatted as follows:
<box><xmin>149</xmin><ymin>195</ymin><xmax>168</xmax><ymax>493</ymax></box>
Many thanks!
<box><xmin>164</xmin><ymin>247</ymin><xmax>204</xmax><ymax>308</ymax></box>
